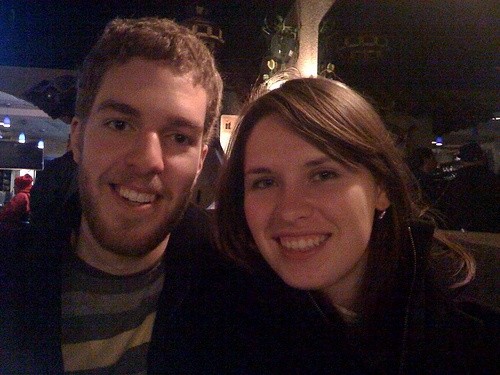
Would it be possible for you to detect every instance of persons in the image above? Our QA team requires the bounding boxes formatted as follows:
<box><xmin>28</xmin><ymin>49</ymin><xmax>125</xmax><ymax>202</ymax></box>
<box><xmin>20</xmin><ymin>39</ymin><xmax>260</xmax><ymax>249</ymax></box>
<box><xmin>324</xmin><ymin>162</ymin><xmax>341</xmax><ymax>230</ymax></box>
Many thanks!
<box><xmin>399</xmin><ymin>148</ymin><xmax>436</xmax><ymax>199</ymax></box>
<box><xmin>433</xmin><ymin>144</ymin><xmax>500</xmax><ymax>227</ymax></box>
<box><xmin>0</xmin><ymin>16</ymin><xmax>500</xmax><ymax>375</ymax></box>
<box><xmin>0</xmin><ymin>173</ymin><xmax>35</xmax><ymax>222</ymax></box>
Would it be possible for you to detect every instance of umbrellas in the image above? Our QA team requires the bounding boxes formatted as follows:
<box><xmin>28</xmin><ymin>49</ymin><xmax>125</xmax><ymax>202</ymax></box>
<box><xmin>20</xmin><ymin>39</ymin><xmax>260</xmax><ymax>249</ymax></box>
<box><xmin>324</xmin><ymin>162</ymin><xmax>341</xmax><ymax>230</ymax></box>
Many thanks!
<box><xmin>0</xmin><ymin>90</ymin><xmax>75</xmax><ymax>161</ymax></box>
